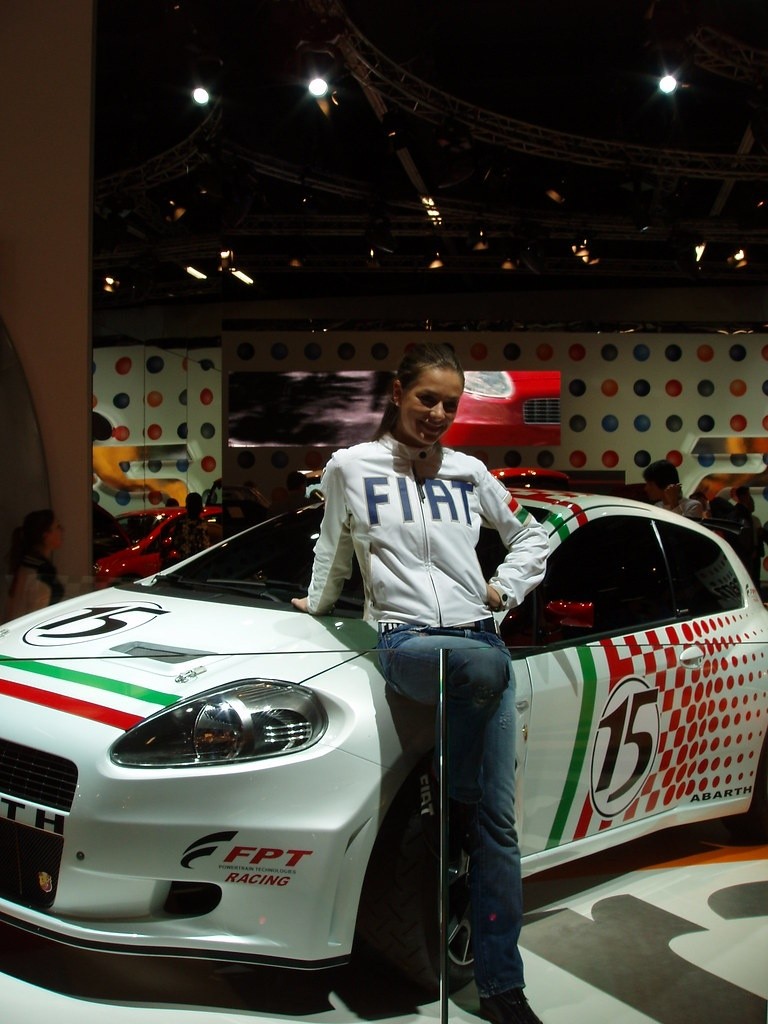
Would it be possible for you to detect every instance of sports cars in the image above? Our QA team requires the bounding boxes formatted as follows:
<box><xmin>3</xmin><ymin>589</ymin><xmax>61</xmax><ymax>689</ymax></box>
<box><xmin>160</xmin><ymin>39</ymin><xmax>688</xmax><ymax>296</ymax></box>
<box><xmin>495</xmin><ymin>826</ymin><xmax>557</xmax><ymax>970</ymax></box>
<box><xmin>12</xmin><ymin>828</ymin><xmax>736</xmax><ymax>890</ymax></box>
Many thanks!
<box><xmin>0</xmin><ymin>483</ymin><xmax>767</xmax><ymax>999</ymax></box>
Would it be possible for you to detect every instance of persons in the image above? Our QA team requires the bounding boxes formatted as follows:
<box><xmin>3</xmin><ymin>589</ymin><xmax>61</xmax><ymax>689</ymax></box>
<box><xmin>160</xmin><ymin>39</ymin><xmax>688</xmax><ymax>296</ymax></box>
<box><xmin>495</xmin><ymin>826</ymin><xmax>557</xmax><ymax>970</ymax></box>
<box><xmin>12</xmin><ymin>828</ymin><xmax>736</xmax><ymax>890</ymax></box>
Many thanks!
<box><xmin>285</xmin><ymin>471</ymin><xmax>313</xmax><ymax>511</ymax></box>
<box><xmin>643</xmin><ymin>459</ymin><xmax>703</xmax><ymax>524</ymax></box>
<box><xmin>294</xmin><ymin>344</ymin><xmax>551</xmax><ymax>1023</ymax></box>
<box><xmin>176</xmin><ymin>492</ymin><xmax>210</xmax><ymax>560</ymax></box>
<box><xmin>1</xmin><ymin>509</ymin><xmax>64</xmax><ymax>627</ymax></box>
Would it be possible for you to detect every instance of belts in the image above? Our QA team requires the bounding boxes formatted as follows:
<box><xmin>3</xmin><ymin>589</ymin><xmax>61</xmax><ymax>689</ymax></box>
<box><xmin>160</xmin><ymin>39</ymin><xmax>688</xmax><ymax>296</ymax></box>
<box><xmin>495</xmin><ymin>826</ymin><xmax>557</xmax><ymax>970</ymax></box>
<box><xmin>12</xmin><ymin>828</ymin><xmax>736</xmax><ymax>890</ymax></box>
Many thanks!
<box><xmin>452</xmin><ymin>617</ymin><xmax>495</xmax><ymax>632</ymax></box>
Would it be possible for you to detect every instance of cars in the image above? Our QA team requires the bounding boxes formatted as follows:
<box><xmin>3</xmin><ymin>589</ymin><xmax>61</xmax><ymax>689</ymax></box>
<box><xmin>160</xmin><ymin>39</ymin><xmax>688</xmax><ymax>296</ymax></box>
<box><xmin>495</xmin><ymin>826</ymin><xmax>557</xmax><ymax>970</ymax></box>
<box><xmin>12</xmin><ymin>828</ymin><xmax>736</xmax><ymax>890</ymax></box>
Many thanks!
<box><xmin>485</xmin><ymin>466</ymin><xmax>575</xmax><ymax>492</ymax></box>
<box><xmin>92</xmin><ymin>502</ymin><xmax>236</xmax><ymax>599</ymax></box>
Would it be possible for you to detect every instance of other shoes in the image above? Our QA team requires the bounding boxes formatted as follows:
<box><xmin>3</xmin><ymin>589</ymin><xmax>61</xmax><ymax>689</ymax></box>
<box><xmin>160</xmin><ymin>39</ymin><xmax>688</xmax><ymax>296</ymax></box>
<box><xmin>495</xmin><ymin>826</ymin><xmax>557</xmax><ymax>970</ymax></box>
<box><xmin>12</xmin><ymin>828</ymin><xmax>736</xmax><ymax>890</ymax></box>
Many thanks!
<box><xmin>479</xmin><ymin>986</ymin><xmax>544</xmax><ymax>1024</ymax></box>
<box><xmin>407</xmin><ymin>745</ymin><xmax>477</xmax><ymax>865</ymax></box>
